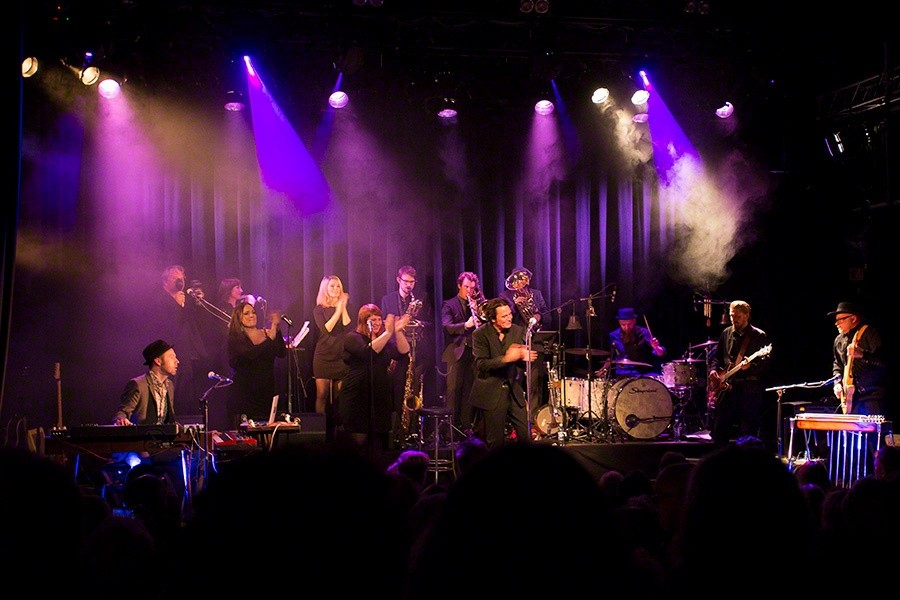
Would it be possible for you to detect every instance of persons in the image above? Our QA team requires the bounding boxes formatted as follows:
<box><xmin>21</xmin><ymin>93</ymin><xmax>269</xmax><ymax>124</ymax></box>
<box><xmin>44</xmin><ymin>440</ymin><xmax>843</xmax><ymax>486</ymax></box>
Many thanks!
<box><xmin>151</xmin><ymin>264</ymin><xmax>213</xmax><ymax>416</ymax></box>
<box><xmin>1</xmin><ymin>434</ymin><xmax>900</xmax><ymax>600</ymax></box>
<box><xmin>378</xmin><ymin>265</ymin><xmax>434</xmax><ymax>434</ymax></box>
<box><xmin>313</xmin><ymin>273</ymin><xmax>355</xmax><ymax>413</ymax></box>
<box><xmin>594</xmin><ymin>307</ymin><xmax>667</xmax><ymax>379</ymax></box>
<box><xmin>216</xmin><ymin>279</ymin><xmax>245</xmax><ymax>339</ymax></box>
<box><xmin>113</xmin><ymin>339</ymin><xmax>207</xmax><ymax>526</ymax></box>
<box><xmin>471</xmin><ymin>297</ymin><xmax>537</xmax><ymax>442</ymax></box>
<box><xmin>828</xmin><ymin>300</ymin><xmax>886</xmax><ymax>416</ymax></box>
<box><xmin>227</xmin><ymin>302</ymin><xmax>287</xmax><ymax>431</ymax></box>
<box><xmin>344</xmin><ymin>303</ymin><xmax>411</xmax><ymax>452</ymax></box>
<box><xmin>442</xmin><ymin>272</ymin><xmax>485</xmax><ymax>439</ymax></box>
<box><xmin>498</xmin><ymin>265</ymin><xmax>553</xmax><ymax>425</ymax></box>
<box><xmin>709</xmin><ymin>300</ymin><xmax>773</xmax><ymax>430</ymax></box>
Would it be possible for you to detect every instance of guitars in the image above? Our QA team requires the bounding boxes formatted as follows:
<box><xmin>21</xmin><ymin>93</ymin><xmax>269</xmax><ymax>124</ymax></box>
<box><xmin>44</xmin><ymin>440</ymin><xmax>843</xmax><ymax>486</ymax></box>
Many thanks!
<box><xmin>706</xmin><ymin>342</ymin><xmax>773</xmax><ymax>408</ymax></box>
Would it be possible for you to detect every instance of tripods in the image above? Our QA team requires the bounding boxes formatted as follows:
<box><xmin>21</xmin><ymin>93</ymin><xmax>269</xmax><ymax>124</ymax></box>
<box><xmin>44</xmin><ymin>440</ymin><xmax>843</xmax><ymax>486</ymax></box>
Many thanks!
<box><xmin>544</xmin><ymin>295</ymin><xmax>625</xmax><ymax>445</ymax></box>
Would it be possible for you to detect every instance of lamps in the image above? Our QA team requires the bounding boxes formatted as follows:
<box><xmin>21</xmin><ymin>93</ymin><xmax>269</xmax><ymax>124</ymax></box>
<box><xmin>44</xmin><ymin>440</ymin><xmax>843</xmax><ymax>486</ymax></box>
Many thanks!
<box><xmin>13</xmin><ymin>48</ymin><xmax>737</xmax><ymax>120</ymax></box>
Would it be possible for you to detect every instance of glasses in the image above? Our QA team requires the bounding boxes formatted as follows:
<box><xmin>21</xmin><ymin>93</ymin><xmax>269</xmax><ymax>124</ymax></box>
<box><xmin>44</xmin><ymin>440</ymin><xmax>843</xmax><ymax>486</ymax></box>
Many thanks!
<box><xmin>400</xmin><ymin>277</ymin><xmax>415</xmax><ymax>283</ymax></box>
<box><xmin>833</xmin><ymin>315</ymin><xmax>853</xmax><ymax>323</ymax></box>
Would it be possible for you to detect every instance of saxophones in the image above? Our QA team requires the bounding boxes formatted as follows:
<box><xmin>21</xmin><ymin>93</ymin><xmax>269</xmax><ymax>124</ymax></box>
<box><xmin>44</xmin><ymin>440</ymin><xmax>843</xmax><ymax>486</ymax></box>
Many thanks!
<box><xmin>398</xmin><ymin>356</ymin><xmax>423</xmax><ymax>452</ymax></box>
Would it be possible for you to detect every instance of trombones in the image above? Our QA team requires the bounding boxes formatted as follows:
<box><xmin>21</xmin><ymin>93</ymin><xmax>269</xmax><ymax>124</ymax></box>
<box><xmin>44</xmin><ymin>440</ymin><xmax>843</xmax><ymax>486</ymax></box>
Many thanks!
<box><xmin>465</xmin><ymin>284</ymin><xmax>490</xmax><ymax>329</ymax></box>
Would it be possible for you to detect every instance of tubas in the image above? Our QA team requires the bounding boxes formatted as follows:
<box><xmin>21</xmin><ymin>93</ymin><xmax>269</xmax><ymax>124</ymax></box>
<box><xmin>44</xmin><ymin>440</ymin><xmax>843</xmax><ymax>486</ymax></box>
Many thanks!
<box><xmin>504</xmin><ymin>270</ymin><xmax>543</xmax><ymax>333</ymax></box>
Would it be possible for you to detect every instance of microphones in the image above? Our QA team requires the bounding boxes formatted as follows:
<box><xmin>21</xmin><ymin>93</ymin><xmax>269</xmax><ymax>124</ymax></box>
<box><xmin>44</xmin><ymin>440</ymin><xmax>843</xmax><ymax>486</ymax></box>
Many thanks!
<box><xmin>366</xmin><ymin>320</ymin><xmax>373</xmax><ymax>332</ymax></box>
<box><xmin>836</xmin><ymin>374</ymin><xmax>841</xmax><ymax>382</ymax></box>
<box><xmin>529</xmin><ymin>313</ymin><xmax>541</xmax><ymax>324</ymax></box>
<box><xmin>187</xmin><ymin>288</ymin><xmax>196</xmax><ymax>297</ymax></box>
<box><xmin>208</xmin><ymin>372</ymin><xmax>232</xmax><ymax>382</ymax></box>
<box><xmin>257</xmin><ymin>296</ymin><xmax>266</xmax><ymax>303</ymax></box>
<box><xmin>611</xmin><ymin>285</ymin><xmax>616</xmax><ymax>302</ymax></box>
<box><xmin>628</xmin><ymin>417</ymin><xmax>639</xmax><ymax>423</ymax></box>
<box><xmin>281</xmin><ymin>314</ymin><xmax>293</xmax><ymax>327</ymax></box>
<box><xmin>693</xmin><ymin>296</ymin><xmax>697</xmax><ymax>312</ymax></box>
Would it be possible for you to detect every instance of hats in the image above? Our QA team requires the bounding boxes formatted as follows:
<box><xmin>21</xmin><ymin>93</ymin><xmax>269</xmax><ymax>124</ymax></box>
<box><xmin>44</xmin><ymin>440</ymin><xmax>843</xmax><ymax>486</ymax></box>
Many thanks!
<box><xmin>616</xmin><ymin>307</ymin><xmax>638</xmax><ymax>320</ymax></box>
<box><xmin>143</xmin><ymin>339</ymin><xmax>174</xmax><ymax>365</ymax></box>
<box><xmin>827</xmin><ymin>302</ymin><xmax>868</xmax><ymax>316</ymax></box>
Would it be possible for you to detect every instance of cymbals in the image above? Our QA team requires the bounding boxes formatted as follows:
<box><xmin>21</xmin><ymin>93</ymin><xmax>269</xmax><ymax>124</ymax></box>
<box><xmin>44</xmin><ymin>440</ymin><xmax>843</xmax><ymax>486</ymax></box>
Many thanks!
<box><xmin>670</xmin><ymin>357</ymin><xmax>706</xmax><ymax>363</ymax></box>
<box><xmin>688</xmin><ymin>340</ymin><xmax>718</xmax><ymax>351</ymax></box>
<box><xmin>564</xmin><ymin>347</ymin><xmax>611</xmax><ymax>356</ymax></box>
<box><xmin>600</xmin><ymin>358</ymin><xmax>654</xmax><ymax>367</ymax></box>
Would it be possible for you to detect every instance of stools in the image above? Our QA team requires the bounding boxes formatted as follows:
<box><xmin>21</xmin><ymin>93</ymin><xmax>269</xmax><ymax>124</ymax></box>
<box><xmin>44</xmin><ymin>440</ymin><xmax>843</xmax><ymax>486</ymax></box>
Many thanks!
<box><xmin>415</xmin><ymin>409</ymin><xmax>459</xmax><ymax>485</ymax></box>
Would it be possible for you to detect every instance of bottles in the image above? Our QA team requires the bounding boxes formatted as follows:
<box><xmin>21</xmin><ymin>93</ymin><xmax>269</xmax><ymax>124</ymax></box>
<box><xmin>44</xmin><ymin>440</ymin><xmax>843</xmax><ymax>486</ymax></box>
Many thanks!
<box><xmin>48</xmin><ymin>424</ymin><xmax>68</xmax><ymax>457</ymax></box>
<box><xmin>240</xmin><ymin>413</ymin><xmax>248</xmax><ymax>430</ymax></box>
<box><xmin>557</xmin><ymin>423</ymin><xmax>565</xmax><ymax>446</ymax></box>
<box><xmin>673</xmin><ymin>419</ymin><xmax>680</xmax><ymax>442</ymax></box>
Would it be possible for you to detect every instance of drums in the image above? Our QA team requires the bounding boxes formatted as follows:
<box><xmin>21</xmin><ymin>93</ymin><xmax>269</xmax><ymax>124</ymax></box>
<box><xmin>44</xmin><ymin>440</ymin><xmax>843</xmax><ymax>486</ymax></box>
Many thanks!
<box><xmin>531</xmin><ymin>402</ymin><xmax>565</xmax><ymax>438</ymax></box>
<box><xmin>660</xmin><ymin>362</ymin><xmax>699</xmax><ymax>392</ymax></box>
<box><xmin>549</xmin><ymin>375</ymin><xmax>674</xmax><ymax>441</ymax></box>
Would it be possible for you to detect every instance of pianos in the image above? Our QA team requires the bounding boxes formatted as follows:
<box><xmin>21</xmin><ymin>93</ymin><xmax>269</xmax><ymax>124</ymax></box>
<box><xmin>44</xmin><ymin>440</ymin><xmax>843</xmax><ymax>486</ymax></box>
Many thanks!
<box><xmin>51</xmin><ymin>424</ymin><xmax>207</xmax><ymax>508</ymax></box>
<box><xmin>208</xmin><ymin>429</ymin><xmax>265</xmax><ymax>471</ymax></box>
<box><xmin>786</xmin><ymin>412</ymin><xmax>893</xmax><ymax>488</ymax></box>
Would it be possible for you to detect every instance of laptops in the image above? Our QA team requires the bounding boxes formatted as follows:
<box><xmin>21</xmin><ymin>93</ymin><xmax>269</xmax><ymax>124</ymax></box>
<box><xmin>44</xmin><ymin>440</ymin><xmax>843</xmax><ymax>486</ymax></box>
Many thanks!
<box><xmin>240</xmin><ymin>394</ymin><xmax>279</xmax><ymax>426</ymax></box>
